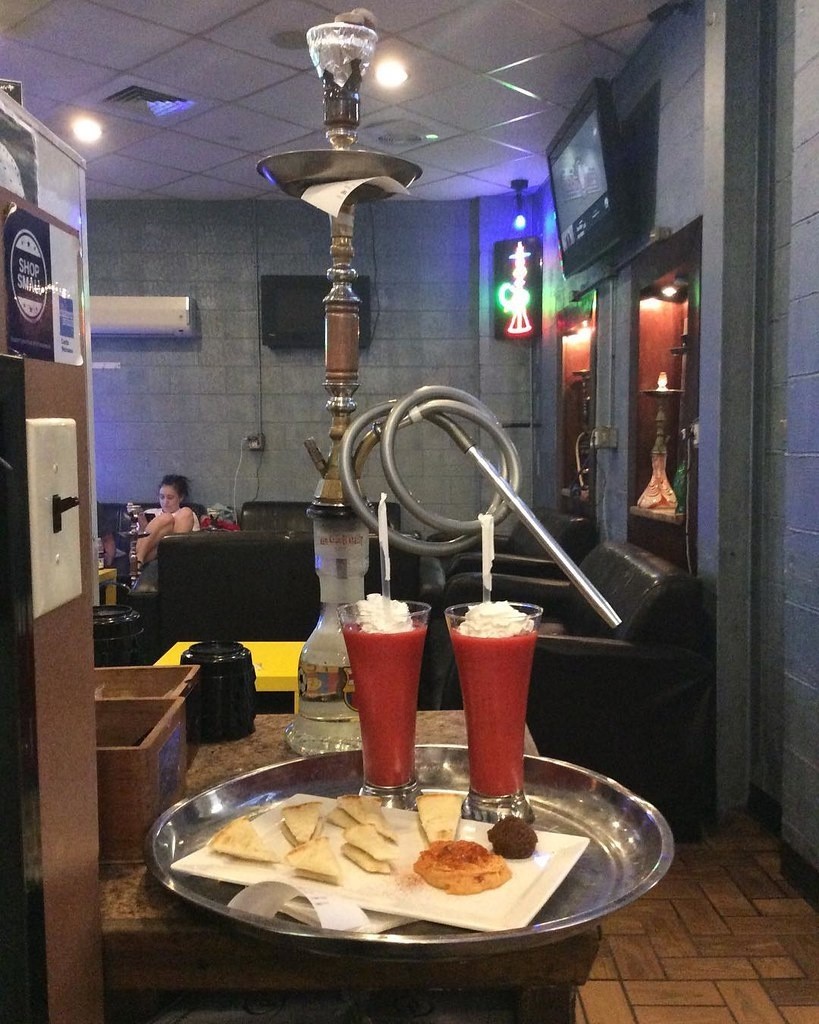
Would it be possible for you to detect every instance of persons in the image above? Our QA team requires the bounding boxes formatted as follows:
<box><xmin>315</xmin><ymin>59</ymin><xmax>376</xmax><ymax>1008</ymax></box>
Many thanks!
<box><xmin>125</xmin><ymin>473</ymin><xmax>202</xmax><ymax>582</ymax></box>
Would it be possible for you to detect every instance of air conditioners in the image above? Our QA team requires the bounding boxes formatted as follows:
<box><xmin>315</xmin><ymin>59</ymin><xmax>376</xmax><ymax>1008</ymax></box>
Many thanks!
<box><xmin>90</xmin><ymin>295</ymin><xmax>199</xmax><ymax>339</ymax></box>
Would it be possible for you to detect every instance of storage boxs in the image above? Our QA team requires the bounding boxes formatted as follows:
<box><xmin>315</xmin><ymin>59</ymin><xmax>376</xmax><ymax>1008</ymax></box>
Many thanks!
<box><xmin>94</xmin><ymin>665</ymin><xmax>200</xmax><ymax>771</ymax></box>
<box><xmin>97</xmin><ymin>697</ymin><xmax>185</xmax><ymax>862</ymax></box>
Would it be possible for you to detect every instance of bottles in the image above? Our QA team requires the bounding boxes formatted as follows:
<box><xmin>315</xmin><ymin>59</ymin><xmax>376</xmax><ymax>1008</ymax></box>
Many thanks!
<box><xmin>98</xmin><ymin>538</ymin><xmax>104</xmax><ymax>570</ymax></box>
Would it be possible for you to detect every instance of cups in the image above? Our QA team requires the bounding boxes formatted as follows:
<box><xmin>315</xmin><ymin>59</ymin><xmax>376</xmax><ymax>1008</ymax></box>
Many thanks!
<box><xmin>93</xmin><ymin>605</ymin><xmax>146</xmax><ymax>668</ymax></box>
<box><xmin>180</xmin><ymin>639</ymin><xmax>258</xmax><ymax>743</ymax></box>
<box><xmin>444</xmin><ymin>601</ymin><xmax>543</xmax><ymax>825</ymax></box>
<box><xmin>336</xmin><ymin>598</ymin><xmax>431</xmax><ymax>811</ymax></box>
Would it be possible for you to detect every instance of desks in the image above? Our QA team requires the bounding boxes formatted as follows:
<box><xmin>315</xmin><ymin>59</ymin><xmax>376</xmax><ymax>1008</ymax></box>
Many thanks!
<box><xmin>152</xmin><ymin>641</ymin><xmax>306</xmax><ymax>714</ymax></box>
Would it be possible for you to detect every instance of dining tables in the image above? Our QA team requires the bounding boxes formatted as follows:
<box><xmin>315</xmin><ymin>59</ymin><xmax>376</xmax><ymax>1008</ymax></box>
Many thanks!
<box><xmin>103</xmin><ymin>711</ymin><xmax>600</xmax><ymax>1024</ymax></box>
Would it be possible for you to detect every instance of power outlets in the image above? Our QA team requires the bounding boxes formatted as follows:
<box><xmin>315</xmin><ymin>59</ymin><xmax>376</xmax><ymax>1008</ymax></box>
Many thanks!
<box><xmin>248</xmin><ymin>433</ymin><xmax>266</xmax><ymax>451</ymax></box>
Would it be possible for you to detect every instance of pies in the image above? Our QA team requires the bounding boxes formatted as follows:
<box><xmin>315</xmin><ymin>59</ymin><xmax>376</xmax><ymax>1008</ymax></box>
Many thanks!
<box><xmin>208</xmin><ymin>794</ymin><xmax>464</xmax><ymax>884</ymax></box>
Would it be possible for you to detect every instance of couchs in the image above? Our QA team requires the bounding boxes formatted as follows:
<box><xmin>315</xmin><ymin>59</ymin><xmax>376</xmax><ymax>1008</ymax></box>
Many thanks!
<box><xmin>240</xmin><ymin>501</ymin><xmax>402</xmax><ymax>530</ymax></box>
<box><xmin>97</xmin><ymin>502</ymin><xmax>206</xmax><ymax>582</ymax></box>
<box><xmin>159</xmin><ymin>527</ymin><xmax>420</xmax><ymax>658</ymax></box>
<box><xmin>426</xmin><ymin>508</ymin><xmax>589</xmax><ymax>581</ymax></box>
<box><xmin>446</xmin><ymin>542</ymin><xmax>704</xmax><ymax>844</ymax></box>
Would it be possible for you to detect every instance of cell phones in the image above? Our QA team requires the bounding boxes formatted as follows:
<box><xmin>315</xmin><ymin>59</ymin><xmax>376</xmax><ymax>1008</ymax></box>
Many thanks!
<box><xmin>145</xmin><ymin>513</ymin><xmax>155</xmax><ymax>522</ymax></box>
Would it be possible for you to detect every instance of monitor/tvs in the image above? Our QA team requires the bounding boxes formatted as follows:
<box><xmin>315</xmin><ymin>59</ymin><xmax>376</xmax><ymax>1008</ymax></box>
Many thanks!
<box><xmin>260</xmin><ymin>275</ymin><xmax>371</xmax><ymax>349</ymax></box>
<box><xmin>546</xmin><ymin>75</ymin><xmax>639</xmax><ymax>280</ymax></box>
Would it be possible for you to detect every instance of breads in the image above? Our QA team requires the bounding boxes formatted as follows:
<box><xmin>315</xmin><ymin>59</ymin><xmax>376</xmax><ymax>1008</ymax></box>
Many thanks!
<box><xmin>487</xmin><ymin>815</ymin><xmax>537</xmax><ymax>859</ymax></box>
